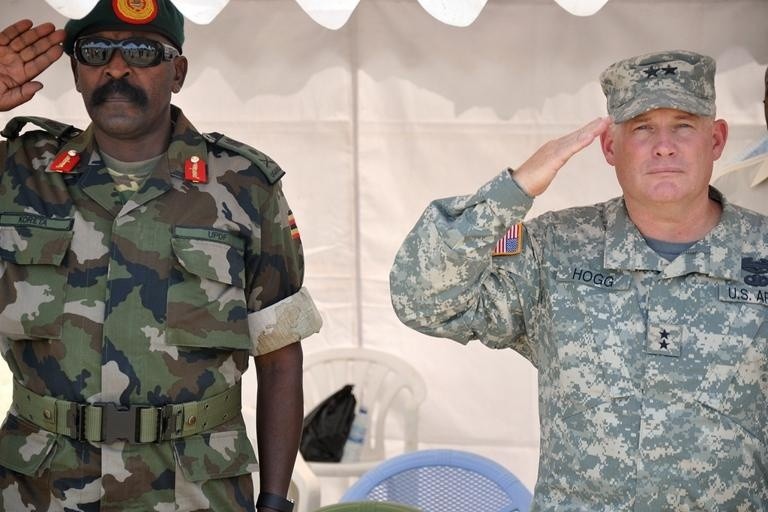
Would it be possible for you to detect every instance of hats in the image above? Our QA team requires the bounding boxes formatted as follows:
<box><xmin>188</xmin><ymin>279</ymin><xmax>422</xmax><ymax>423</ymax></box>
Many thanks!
<box><xmin>59</xmin><ymin>1</ymin><xmax>187</xmax><ymax>57</ymax></box>
<box><xmin>597</xmin><ymin>49</ymin><xmax>720</xmax><ymax>127</ymax></box>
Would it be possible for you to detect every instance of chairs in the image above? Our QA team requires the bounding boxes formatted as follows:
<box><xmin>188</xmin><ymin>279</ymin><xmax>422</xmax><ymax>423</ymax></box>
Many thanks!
<box><xmin>287</xmin><ymin>346</ymin><xmax>427</xmax><ymax>512</ymax></box>
<box><xmin>338</xmin><ymin>448</ymin><xmax>534</xmax><ymax>512</ymax></box>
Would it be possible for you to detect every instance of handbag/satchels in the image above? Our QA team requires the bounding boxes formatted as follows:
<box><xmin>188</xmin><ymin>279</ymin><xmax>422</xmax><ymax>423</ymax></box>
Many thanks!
<box><xmin>300</xmin><ymin>385</ymin><xmax>356</xmax><ymax>463</ymax></box>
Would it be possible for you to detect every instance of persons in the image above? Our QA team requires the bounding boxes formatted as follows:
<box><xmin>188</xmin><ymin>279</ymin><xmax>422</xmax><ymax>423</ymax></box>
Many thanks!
<box><xmin>1</xmin><ymin>1</ymin><xmax>322</xmax><ymax>511</ymax></box>
<box><xmin>389</xmin><ymin>46</ymin><xmax>766</xmax><ymax>511</ymax></box>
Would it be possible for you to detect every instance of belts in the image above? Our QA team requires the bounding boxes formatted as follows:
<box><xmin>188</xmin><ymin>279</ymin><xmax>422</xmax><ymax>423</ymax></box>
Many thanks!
<box><xmin>13</xmin><ymin>376</ymin><xmax>242</xmax><ymax>446</ymax></box>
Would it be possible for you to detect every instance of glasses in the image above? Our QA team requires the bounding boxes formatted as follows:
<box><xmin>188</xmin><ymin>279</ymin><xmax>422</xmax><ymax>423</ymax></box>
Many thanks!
<box><xmin>69</xmin><ymin>33</ymin><xmax>179</xmax><ymax>71</ymax></box>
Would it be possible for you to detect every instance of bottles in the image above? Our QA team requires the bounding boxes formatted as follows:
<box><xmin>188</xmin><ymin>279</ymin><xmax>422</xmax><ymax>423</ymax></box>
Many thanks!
<box><xmin>341</xmin><ymin>407</ymin><xmax>368</xmax><ymax>463</ymax></box>
